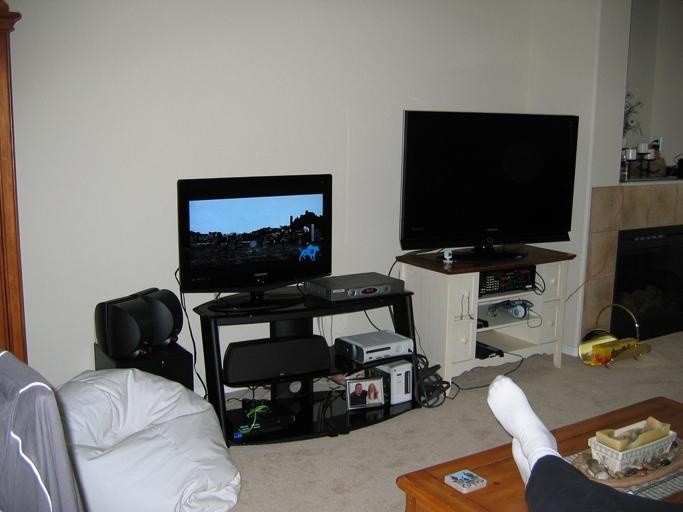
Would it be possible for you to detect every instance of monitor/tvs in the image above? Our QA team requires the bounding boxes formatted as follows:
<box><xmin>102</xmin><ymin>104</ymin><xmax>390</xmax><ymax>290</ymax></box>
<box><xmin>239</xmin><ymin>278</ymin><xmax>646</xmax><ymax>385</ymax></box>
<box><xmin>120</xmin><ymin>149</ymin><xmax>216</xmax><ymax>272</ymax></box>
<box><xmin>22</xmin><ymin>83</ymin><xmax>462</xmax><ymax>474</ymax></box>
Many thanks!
<box><xmin>398</xmin><ymin>107</ymin><xmax>581</xmax><ymax>259</ymax></box>
<box><xmin>177</xmin><ymin>173</ymin><xmax>333</xmax><ymax>307</ymax></box>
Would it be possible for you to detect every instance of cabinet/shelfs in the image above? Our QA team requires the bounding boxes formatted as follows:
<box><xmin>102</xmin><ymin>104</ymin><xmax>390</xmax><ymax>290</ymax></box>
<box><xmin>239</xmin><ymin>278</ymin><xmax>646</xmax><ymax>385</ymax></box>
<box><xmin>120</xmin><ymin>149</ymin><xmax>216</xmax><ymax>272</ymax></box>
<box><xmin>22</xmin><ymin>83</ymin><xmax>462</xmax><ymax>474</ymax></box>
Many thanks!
<box><xmin>396</xmin><ymin>244</ymin><xmax>576</xmax><ymax>397</ymax></box>
<box><xmin>192</xmin><ymin>286</ymin><xmax>421</xmax><ymax>448</ymax></box>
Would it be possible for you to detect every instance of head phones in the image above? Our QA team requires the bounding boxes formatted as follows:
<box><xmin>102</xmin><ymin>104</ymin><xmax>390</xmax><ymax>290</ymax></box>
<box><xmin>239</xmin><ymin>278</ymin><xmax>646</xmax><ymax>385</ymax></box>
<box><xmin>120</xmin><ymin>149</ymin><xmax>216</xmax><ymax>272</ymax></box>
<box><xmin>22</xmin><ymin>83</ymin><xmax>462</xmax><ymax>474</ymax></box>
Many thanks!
<box><xmin>500</xmin><ymin>299</ymin><xmax>533</xmax><ymax>319</ymax></box>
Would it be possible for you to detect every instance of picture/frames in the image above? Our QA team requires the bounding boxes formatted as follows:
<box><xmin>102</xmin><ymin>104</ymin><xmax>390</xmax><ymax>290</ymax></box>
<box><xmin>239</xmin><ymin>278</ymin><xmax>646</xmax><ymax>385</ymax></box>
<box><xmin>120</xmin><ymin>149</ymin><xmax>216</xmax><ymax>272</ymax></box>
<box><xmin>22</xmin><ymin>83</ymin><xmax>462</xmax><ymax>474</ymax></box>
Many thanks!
<box><xmin>346</xmin><ymin>376</ymin><xmax>385</xmax><ymax>411</ymax></box>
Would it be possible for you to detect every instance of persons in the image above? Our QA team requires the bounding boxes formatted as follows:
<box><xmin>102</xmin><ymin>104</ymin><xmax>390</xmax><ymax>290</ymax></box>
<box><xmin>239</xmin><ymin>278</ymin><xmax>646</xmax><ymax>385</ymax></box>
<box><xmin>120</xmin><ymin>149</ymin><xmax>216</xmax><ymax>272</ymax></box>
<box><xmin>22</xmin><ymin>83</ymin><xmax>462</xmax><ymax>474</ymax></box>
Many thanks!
<box><xmin>365</xmin><ymin>383</ymin><xmax>380</xmax><ymax>403</ymax></box>
<box><xmin>348</xmin><ymin>383</ymin><xmax>368</xmax><ymax>406</ymax></box>
<box><xmin>487</xmin><ymin>374</ymin><xmax>683</xmax><ymax>512</ymax></box>
